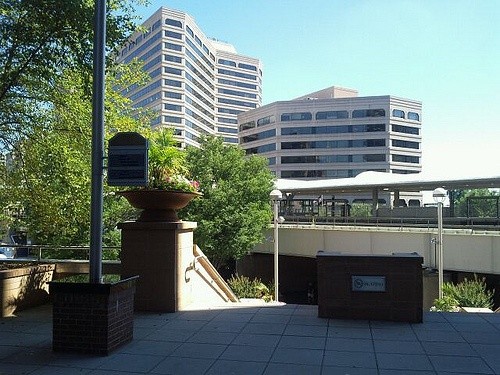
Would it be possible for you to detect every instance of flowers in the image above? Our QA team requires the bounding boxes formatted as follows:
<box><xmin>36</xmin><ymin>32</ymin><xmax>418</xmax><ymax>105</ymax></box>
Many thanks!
<box><xmin>118</xmin><ymin>170</ymin><xmax>204</xmax><ymax>194</ymax></box>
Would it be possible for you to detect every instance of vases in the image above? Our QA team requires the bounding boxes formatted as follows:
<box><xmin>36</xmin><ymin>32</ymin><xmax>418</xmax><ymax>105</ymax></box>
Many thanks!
<box><xmin>119</xmin><ymin>186</ymin><xmax>202</xmax><ymax>223</ymax></box>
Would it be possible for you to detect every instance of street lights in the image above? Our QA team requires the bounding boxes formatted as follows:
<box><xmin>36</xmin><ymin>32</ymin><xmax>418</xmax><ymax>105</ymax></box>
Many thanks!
<box><xmin>432</xmin><ymin>187</ymin><xmax>447</xmax><ymax>301</ymax></box>
<box><xmin>270</xmin><ymin>190</ymin><xmax>285</xmax><ymax>301</ymax></box>
